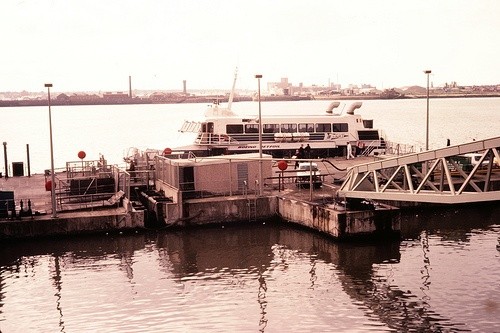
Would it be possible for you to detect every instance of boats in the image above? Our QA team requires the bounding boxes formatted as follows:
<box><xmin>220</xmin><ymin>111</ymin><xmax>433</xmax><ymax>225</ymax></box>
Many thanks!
<box><xmin>122</xmin><ymin>102</ymin><xmax>385</xmax><ymax>167</ymax></box>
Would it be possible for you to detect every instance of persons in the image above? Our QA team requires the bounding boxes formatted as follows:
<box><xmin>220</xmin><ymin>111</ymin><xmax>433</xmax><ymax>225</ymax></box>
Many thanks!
<box><xmin>304</xmin><ymin>144</ymin><xmax>311</xmax><ymax>159</ymax></box>
<box><xmin>294</xmin><ymin>150</ymin><xmax>299</xmax><ymax>169</ymax></box>
<box><xmin>299</xmin><ymin>144</ymin><xmax>305</xmax><ymax>159</ymax></box>
<box><xmin>347</xmin><ymin>142</ymin><xmax>355</xmax><ymax>160</ymax></box>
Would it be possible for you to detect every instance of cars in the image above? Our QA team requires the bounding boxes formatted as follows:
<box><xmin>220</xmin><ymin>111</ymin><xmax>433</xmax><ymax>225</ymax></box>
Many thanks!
<box><xmin>295</xmin><ymin>163</ymin><xmax>322</xmax><ymax>186</ymax></box>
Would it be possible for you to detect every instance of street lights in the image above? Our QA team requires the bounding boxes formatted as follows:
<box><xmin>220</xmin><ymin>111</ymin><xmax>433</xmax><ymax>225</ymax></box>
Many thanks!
<box><xmin>45</xmin><ymin>84</ymin><xmax>57</xmax><ymax>218</ymax></box>
<box><xmin>256</xmin><ymin>75</ymin><xmax>265</xmax><ymax>196</ymax></box>
<box><xmin>424</xmin><ymin>70</ymin><xmax>430</xmax><ymax>150</ymax></box>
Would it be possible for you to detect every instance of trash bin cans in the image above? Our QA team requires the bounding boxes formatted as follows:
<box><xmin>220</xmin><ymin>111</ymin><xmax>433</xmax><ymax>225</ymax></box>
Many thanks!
<box><xmin>11</xmin><ymin>162</ymin><xmax>24</xmax><ymax>176</ymax></box>
